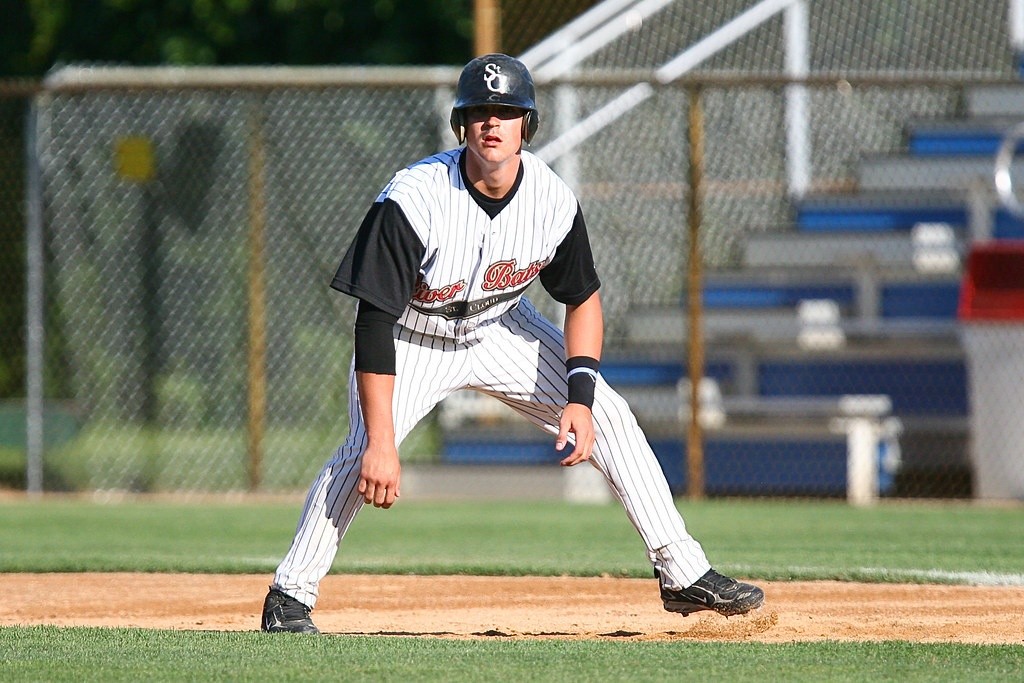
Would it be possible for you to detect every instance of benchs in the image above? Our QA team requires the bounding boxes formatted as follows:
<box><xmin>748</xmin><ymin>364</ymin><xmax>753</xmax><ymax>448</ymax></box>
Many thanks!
<box><xmin>432</xmin><ymin>76</ymin><xmax>1024</xmax><ymax>496</ymax></box>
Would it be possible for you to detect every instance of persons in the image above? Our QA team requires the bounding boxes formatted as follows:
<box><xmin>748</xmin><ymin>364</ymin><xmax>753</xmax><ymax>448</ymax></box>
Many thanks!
<box><xmin>262</xmin><ymin>55</ymin><xmax>762</xmax><ymax>635</ymax></box>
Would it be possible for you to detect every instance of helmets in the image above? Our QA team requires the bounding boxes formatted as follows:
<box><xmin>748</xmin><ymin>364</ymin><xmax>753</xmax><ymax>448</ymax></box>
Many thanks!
<box><xmin>449</xmin><ymin>55</ymin><xmax>539</xmax><ymax>147</ymax></box>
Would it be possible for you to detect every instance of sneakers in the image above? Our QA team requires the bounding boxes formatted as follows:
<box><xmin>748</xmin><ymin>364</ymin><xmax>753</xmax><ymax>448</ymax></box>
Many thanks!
<box><xmin>261</xmin><ymin>588</ymin><xmax>320</xmax><ymax>635</ymax></box>
<box><xmin>658</xmin><ymin>565</ymin><xmax>764</xmax><ymax>618</ymax></box>
